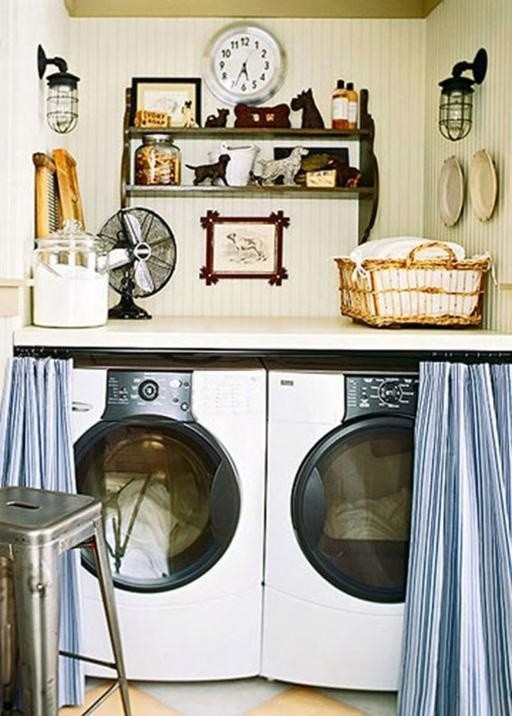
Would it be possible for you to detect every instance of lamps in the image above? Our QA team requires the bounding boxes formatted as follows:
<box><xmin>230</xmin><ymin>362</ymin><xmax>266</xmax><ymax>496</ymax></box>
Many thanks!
<box><xmin>438</xmin><ymin>48</ymin><xmax>487</xmax><ymax>141</ymax></box>
<box><xmin>38</xmin><ymin>44</ymin><xmax>81</xmax><ymax>134</ymax></box>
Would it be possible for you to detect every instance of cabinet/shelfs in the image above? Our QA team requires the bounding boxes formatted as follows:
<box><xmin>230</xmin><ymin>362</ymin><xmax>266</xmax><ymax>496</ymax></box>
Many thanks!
<box><xmin>121</xmin><ymin>87</ymin><xmax>379</xmax><ymax>248</ymax></box>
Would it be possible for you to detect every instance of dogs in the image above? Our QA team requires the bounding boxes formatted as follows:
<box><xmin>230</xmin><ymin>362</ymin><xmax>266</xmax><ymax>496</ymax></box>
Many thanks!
<box><xmin>256</xmin><ymin>146</ymin><xmax>309</xmax><ymax>188</ymax></box>
<box><xmin>182</xmin><ymin>101</ymin><xmax>199</xmax><ymax>129</ymax></box>
<box><xmin>184</xmin><ymin>154</ymin><xmax>231</xmax><ymax>186</ymax></box>
<box><xmin>204</xmin><ymin>108</ymin><xmax>229</xmax><ymax>127</ymax></box>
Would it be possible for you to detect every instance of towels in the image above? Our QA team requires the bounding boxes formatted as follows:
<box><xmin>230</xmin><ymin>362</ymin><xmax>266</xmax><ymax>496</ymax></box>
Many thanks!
<box><xmin>349</xmin><ymin>237</ymin><xmax>464</xmax><ymax>262</ymax></box>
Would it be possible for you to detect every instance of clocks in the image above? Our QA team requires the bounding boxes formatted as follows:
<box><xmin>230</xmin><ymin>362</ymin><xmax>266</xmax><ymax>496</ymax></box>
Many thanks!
<box><xmin>200</xmin><ymin>21</ymin><xmax>288</xmax><ymax>104</ymax></box>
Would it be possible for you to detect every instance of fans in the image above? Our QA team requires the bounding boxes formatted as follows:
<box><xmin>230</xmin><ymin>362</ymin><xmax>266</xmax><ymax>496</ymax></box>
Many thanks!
<box><xmin>97</xmin><ymin>207</ymin><xmax>176</xmax><ymax>319</ymax></box>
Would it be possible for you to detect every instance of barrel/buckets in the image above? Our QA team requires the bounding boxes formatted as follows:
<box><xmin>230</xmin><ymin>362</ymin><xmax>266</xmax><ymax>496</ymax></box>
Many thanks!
<box><xmin>64</xmin><ymin>360</ymin><xmax>267</xmax><ymax>683</ymax></box>
<box><xmin>267</xmin><ymin>364</ymin><xmax>422</xmax><ymax>692</ymax></box>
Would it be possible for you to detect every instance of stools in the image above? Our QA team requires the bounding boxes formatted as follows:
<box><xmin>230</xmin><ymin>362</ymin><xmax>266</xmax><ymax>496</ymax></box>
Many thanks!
<box><xmin>0</xmin><ymin>486</ymin><xmax>131</xmax><ymax>715</ymax></box>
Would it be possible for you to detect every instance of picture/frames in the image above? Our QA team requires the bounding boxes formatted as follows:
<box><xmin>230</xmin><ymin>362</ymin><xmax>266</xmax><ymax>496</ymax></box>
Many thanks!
<box><xmin>130</xmin><ymin>77</ymin><xmax>201</xmax><ymax>129</ymax></box>
<box><xmin>199</xmin><ymin>210</ymin><xmax>290</xmax><ymax>285</ymax></box>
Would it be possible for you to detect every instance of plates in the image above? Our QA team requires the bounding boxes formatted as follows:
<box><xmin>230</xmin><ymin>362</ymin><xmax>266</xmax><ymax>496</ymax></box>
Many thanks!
<box><xmin>435</xmin><ymin>152</ymin><xmax>497</xmax><ymax>228</ymax></box>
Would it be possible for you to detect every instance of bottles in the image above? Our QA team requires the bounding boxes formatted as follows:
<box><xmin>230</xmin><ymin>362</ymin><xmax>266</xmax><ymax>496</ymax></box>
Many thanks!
<box><xmin>132</xmin><ymin>134</ymin><xmax>181</xmax><ymax>186</ymax></box>
<box><xmin>32</xmin><ymin>221</ymin><xmax>106</xmax><ymax>328</ymax></box>
<box><xmin>331</xmin><ymin>78</ymin><xmax>359</xmax><ymax>132</ymax></box>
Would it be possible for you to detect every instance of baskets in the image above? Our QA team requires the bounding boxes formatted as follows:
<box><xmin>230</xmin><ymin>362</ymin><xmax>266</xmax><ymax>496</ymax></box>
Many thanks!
<box><xmin>133</xmin><ymin>110</ymin><xmax>170</xmax><ymax>129</ymax></box>
<box><xmin>306</xmin><ymin>169</ymin><xmax>338</xmax><ymax>188</ymax></box>
